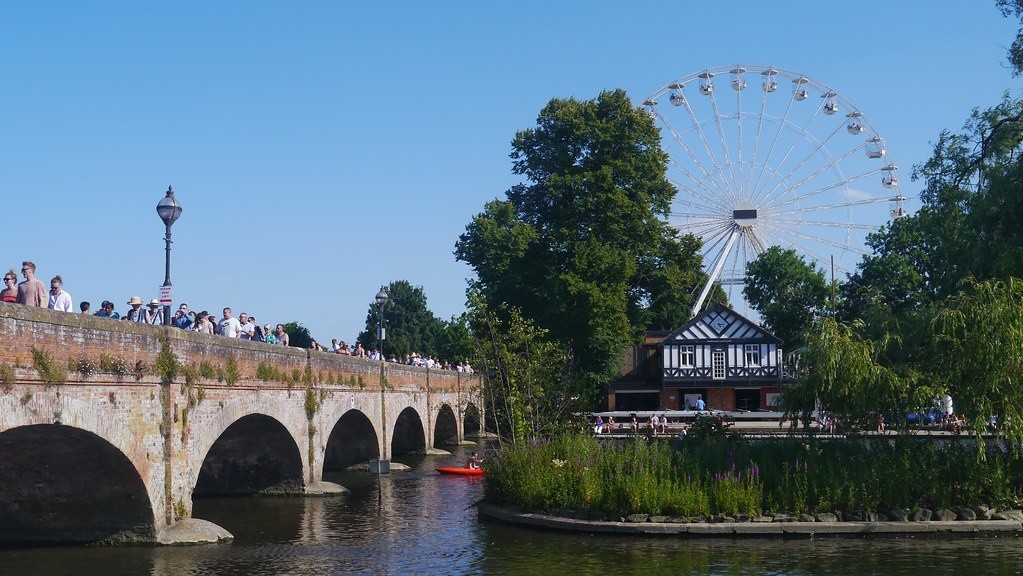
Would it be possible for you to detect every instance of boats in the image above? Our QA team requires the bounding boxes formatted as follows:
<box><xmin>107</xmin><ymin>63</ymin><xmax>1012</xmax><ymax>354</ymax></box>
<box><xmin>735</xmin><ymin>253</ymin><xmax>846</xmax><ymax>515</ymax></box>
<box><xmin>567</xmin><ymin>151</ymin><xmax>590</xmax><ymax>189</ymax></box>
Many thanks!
<box><xmin>434</xmin><ymin>468</ymin><xmax>488</xmax><ymax>475</ymax></box>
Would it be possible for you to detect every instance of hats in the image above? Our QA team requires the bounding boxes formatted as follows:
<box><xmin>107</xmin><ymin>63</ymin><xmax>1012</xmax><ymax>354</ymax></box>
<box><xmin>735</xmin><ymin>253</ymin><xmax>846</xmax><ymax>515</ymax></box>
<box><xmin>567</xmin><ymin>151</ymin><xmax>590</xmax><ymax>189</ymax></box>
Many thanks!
<box><xmin>201</xmin><ymin>311</ymin><xmax>210</xmax><ymax>315</ymax></box>
<box><xmin>127</xmin><ymin>296</ymin><xmax>143</xmax><ymax>305</ymax></box>
<box><xmin>147</xmin><ymin>298</ymin><xmax>159</xmax><ymax>307</ymax></box>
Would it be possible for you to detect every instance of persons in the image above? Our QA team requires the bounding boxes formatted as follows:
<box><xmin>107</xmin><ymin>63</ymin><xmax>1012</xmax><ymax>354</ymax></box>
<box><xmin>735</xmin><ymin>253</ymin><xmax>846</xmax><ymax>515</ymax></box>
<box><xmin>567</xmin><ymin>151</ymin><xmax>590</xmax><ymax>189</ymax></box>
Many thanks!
<box><xmin>80</xmin><ymin>302</ymin><xmax>90</xmax><ymax>313</ymax></box>
<box><xmin>171</xmin><ymin>303</ymin><xmax>217</xmax><ymax>334</ymax></box>
<box><xmin>695</xmin><ymin>396</ymin><xmax>706</xmax><ymax>410</ymax></box>
<box><xmin>0</xmin><ymin>261</ymin><xmax>72</xmax><ymax>311</ymax></box>
<box><xmin>465</xmin><ymin>451</ymin><xmax>484</xmax><ymax>470</ymax></box>
<box><xmin>943</xmin><ymin>410</ymin><xmax>965</xmax><ymax>433</ymax></box>
<box><xmin>817</xmin><ymin>414</ymin><xmax>837</xmax><ymax>433</ymax></box>
<box><xmin>329</xmin><ymin>339</ymin><xmax>385</xmax><ymax>360</ymax></box>
<box><xmin>682</xmin><ymin>399</ymin><xmax>691</xmax><ymax>422</ymax></box>
<box><xmin>878</xmin><ymin>414</ymin><xmax>885</xmax><ymax>432</ymax></box>
<box><xmin>606</xmin><ymin>416</ymin><xmax>615</xmax><ymax>434</ymax></box>
<box><xmin>388</xmin><ymin>351</ymin><xmax>474</xmax><ymax>373</ymax></box>
<box><xmin>121</xmin><ymin>296</ymin><xmax>163</xmax><ymax>324</ymax></box>
<box><xmin>594</xmin><ymin>415</ymin><xmax>603</xmax><ymax>434</ymax></box>
<box><xmin>646</xmin><ymin>413</ymin><xmax>667</xmax><ymax>436</ymax></box>
<box><xmin>680</xmin><ymin>426</ymin><xmax>688</xmax><ymax>440</ymax></box>
<box><xmin>215</xmin><ymin>307</ymin><xmax>289</xmax><ymax>346</ymax></box>
<box><xmin>308</xmin><ymin>340</ymin><xmax>322</xmax><ymax>350</ymax></box>
<box><xmin>629</xmin><ymin>417</ymin><xmax>638</xmax><ymax>433</ymax></box>
<box><xmin>94</xmin><ymin>301</ymin><xmax>119</xmax><ymax>319</ymax></box>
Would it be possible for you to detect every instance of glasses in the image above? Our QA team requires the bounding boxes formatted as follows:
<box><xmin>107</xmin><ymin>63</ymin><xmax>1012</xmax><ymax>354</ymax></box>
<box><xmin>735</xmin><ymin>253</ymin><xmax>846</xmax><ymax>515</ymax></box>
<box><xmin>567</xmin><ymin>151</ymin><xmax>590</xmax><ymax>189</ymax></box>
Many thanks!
<box><xmin>3</xmin><ymin>278</ymin><xmax>13</xmax><ymax>281</ymax></box>
<box><xmin>22</xmin><ymin>268</ymin><xmax>31</xmax><ymax>272</ymax></box>
<box><xmin>51</xmin><ymin>285</ymin><xmax>61</xmax><ymax>290</ymax></box>
<box><xmin>248</xmin><ymin>319</ymin><xmax>252</xmax><ymax>322</ymax></box>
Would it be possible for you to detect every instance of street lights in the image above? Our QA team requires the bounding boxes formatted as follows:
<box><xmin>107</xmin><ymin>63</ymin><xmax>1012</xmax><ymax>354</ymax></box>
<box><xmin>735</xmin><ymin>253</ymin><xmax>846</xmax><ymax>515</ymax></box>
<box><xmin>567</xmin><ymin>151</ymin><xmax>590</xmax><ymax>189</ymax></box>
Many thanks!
<box><xmin>374</xmin><ymin>285</ymin><xmax>390</xmax><ymax>360</ymax></box>
<box><xmin>154</xmin><ymin>184</ymin><xmax>183</xmax><ymax>325</ymax></box>
